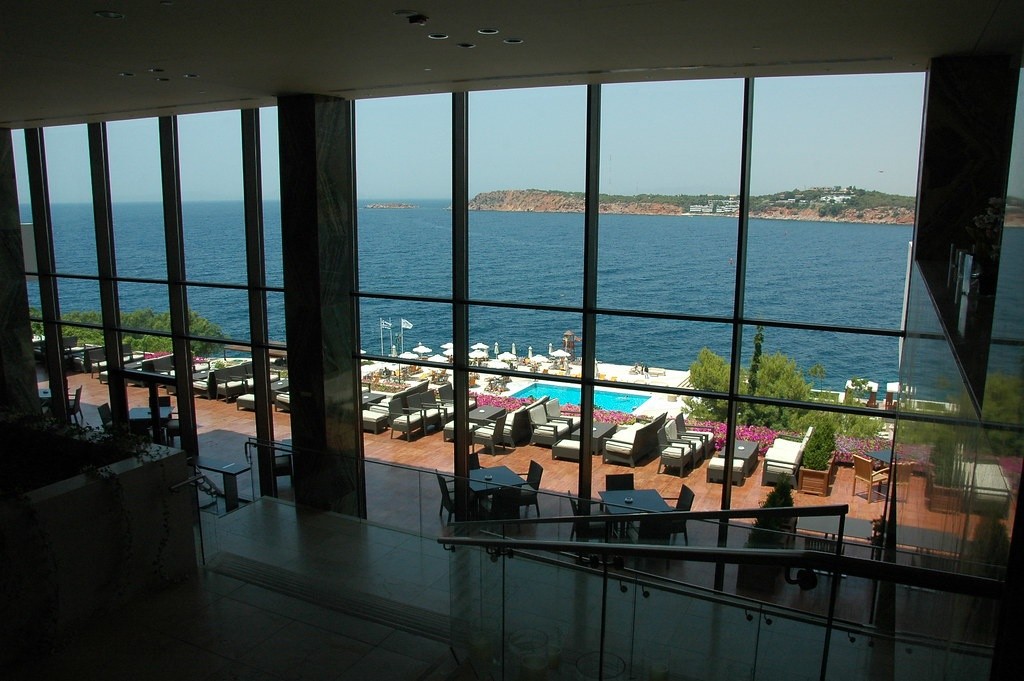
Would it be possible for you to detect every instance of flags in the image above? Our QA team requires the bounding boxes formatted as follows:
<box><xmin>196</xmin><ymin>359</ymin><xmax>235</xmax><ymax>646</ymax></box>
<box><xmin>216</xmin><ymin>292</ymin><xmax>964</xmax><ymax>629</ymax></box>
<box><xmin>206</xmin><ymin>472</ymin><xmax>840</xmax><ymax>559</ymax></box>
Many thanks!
<box><xmin>382</xmin><ymin>320</ymin><xmax>392</xmax><ymax>329</ymax></box>
<box><xmin>402</xmin><ymin>319</ymin><xmax>413</xmax><ymax>329</ymax></box>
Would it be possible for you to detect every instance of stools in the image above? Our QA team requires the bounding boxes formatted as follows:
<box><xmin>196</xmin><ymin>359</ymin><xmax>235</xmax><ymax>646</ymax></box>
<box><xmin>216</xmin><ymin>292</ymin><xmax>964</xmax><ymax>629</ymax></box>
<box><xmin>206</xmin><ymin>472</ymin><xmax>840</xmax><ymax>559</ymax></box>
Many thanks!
<box><xmin>443</xmin><ymin>420</ymin><xmax>454</xmax><ymax>443</ymax></box>
<box><xmin>552</xmin><ymin>438</ymin><xmax>580</xmax><ymax>461</ymax></box>
<box><xmin>708</xmin><ymin>457</ymin><xmax>746</xmax><ymax>487</ymax></box>
<box><xmin>362</xmin><ymin>410</ymin><xmax>388</xmax><ymax>434</ymax></box>
<box><xmin>237</xmin><ymin>393</ymin><xmax>255</xmax><ymax>412</ymax></box>
<box><xmin>275</xmin><ymin>392</ymin><xmax>290</xmax><ymax>413</ymax></box>
<box><xmin>99</xmin><ymin>371</ymin><xmax>109</xmax><ymax>383</ymax></box>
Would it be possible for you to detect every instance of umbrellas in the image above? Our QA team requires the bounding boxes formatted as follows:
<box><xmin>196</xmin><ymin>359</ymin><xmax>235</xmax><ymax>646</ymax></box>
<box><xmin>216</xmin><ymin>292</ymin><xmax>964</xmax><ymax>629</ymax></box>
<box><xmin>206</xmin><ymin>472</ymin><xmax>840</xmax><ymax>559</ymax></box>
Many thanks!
<box><xmin>498</xmin><ymin>351</ymin><xmax>517</xmax><ymax>370</ymax></box>
<box><xmin>398</xmin><ymin>352</ymin><xmax>419</xmax><ymax>372</ymax></box>
<box><xmin>549</xmin><ymin>350</ymin><xmax>571</xmax><ymax>364</ymax></box>
<box><xmin>487</xmin><ymin>359</ymin><xmax>510</xmax><ymax>382</ymax></box>
<box><xmin>548</xmin><ymin>343</ymin><xmax>552</xmax><ymax>355</ymax></box>
<box><xmin>528</xmin><ymin>346</ymin><xmax>533</xmax><ymax>358</ymax></box>
<box><xmin>531</xmin><ymin>354</ymin><xmax>549</xmax><ymax>367</ymax></box>
<box><xmin>511</xmin><ymin>343</ymin><xmax>515</xmax><ymax>355</ymax></box>
<box><xmin>468</xmin><ymin>343</ymin><xmax>489</xmax><ymax>366</ymax></box>
<box><xmin>440</xmin><ymin>343</ymin><xmax>453</xmax><ymax>363</ymax></box>
<box><xmin>494</xmin><ymin>341</ymin><xmax>498</xmax><ymax>354</ymax></box>
<box><xmin>413</xmin><ymin>346</ymin><xmax>432</xmax><ymax>360</ymax></box>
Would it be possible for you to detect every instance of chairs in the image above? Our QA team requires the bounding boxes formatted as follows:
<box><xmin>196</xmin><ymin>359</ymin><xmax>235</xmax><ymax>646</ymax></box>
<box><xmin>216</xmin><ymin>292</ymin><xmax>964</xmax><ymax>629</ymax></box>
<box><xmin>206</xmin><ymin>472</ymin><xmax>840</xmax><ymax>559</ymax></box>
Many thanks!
<box><xmin>472</xmin><ymin>414</ymin><xmax>507</xmax><ymax>456</ymax></box>
<box><xmin>670</xmin><ymin>484</ymin><xmax>695</xmax><ymax>546</ymax></box>
<box><xmin>606</xmin><ymin>474</ymin><xmax>635</xmax><ymax>490</ymax></box>
<box><xmin>517</xmin><ymin>460</ymin><xmax>543</xmax><ymax>518</ymax></box>
<box><xmin>148</xmin><ymin>396</ymin><xmax>180</xmax><ymax>448</ymax></box>
<box><xmin>435</xmin><ymin>469</ymin><xmax>478</xmax><ymax>526</ymax></box>
<box><xmin>468</xmin><ymin>452</ymin><xmax>487</xmax><ymax>470</ymax></box>
<box><xmin>389</xmin><ymin>385</ymin><xmax>461</xmax><ymax>442</ymax></box>
<box><xmin>628</xmin><ymin>512</ymin><xmax>672</xmax><ymax>569</ymax></box>
<box><xmin>98</xmin><ymin>402</ymin><xmax>112</xmax><ymax>428</ymax></box>
<box><xmin>143</xmin><ymin>353</ymin><xmax>210</xmax><ymax>388</ymax></box>
<box><xmin>657</xmin><ymin>413</ymin><xmax>715</xmax><ymax>477</ymax></box>
<box><xmin>88</xmin><ymin>343</ymin><xmax>133</xmax><ymax>379</ymax></box>
<box><xmin>568</xmin><ymin>490</ymin><xmax>611</xmax><ymax>541</ymax></box>
<box><xmin>762</xmin><ymin>424</ymin><xmax>815</xmax><ymax>489</ymax></box>
<box><xmin>527</xmin><ymin>398</ymin><xmax>582</xmax><ymax>449</ymax></box>
<box><xmin>69</xmin><ymin>386</ymin><xmax>83</xmax><ymax>423</ymax></box>
<box><xmin>852</xmin><ymin>454</ymin><xmax>911</xmax><ymax>504</ymax></box>
<box><xmin>215</xmin><ymin>360</ymin><xmax>279</xmax><ymax>404</ymax></box>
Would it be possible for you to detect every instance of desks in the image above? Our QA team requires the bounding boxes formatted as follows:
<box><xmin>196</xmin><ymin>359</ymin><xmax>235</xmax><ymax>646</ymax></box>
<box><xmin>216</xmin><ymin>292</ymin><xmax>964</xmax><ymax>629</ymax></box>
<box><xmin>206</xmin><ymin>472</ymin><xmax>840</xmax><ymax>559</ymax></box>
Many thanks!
<box><xmin>599</xmin><ymin>489</ymin><xmax>673</xmax><ymax>540</ymax></box>
<box><xmin>469</xmin><ymin>466</ymin><xmax>528</xmax><ymax>504</ymax></box>
<box><xmin>470</xmin><ymin>405</ymin><xmax>507</xmax><ymax>425</ymax></box>
<box><xmin>865</xmin><ymin>448</ymin><xmax>904</xmax><ymax>492</ymax></box>
<box><xmin>718</xmin><ymin>440</ymin><xmax>759</xmax><ymax>476</ymax></box>
<box><xmin>362</xmin><ymin>391</ymin><xmax>386</xmax><ymax>408</ymax></box>
<box><xmin>271</xmin><ymin>378</ymin><xmax>288</xmax><ymax>401</ymax></box>
<box><xmin>129</xmin><ymin>407</ymin><xmax>174</xmax><ymax>446</ymax></box>
<box><xmin>66</xmin><ymin>343</ymin><xmax>101</xmax><ymax>354</ymax></box>
<box><xmin>39</xmin><ymin>389</ymin><xmax>55</xmax><ymax>412</ymax></box>
<box><xmin>571</xmin><ymin>420</ymin><xmax>617</xmax><ymax>456</ymax></box>
<box><xmin>192</xmin><ymin>367</ymin><xmax>221</xmax><ymax>380</ymax></box>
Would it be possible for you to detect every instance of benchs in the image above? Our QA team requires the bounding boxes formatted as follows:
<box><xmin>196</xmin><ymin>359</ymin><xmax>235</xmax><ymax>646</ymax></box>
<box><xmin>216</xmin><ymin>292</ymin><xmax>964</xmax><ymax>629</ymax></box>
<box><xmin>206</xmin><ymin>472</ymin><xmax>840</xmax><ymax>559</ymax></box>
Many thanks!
<box><xmin>492</xmin><ymin>399</ymin><xmax>549</xmax><ymax>448</ymax></box>
<box><xmin>603</xmin><ymin>412</ymin><xmax>669</xmax><ymax>467</ymax></box>
<box><xmin>382</xmin><ymin>381</ymin><xmax>429</xmax><ymax>413</ymax></box>
<box><xmin>32</xmin><ymin>335</ymin><xmax>77</xmax><ymax>364</ymax></box>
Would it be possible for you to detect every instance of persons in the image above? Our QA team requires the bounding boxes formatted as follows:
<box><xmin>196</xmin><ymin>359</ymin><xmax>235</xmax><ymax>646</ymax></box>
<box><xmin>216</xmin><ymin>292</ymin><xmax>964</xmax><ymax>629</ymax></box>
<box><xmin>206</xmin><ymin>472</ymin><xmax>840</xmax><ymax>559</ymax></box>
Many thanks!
<box><xmin>510</xmin><ymin>363</ymin><xmax>517</xmax><ymax>370</ymax></box>
<box><xmin>449</xmin><ymin>355</ymin><xmax>452</xmax><ymax>363</ymax></box>
<box><xmin>644</xmin><ymin>365</ymin><xmax>649</xmax><ymax>379</ymax></box>
<box><xmin>383</xmin><ymin>367</ymin><xmax>396</xmax><ymax>380</ymax></box>
